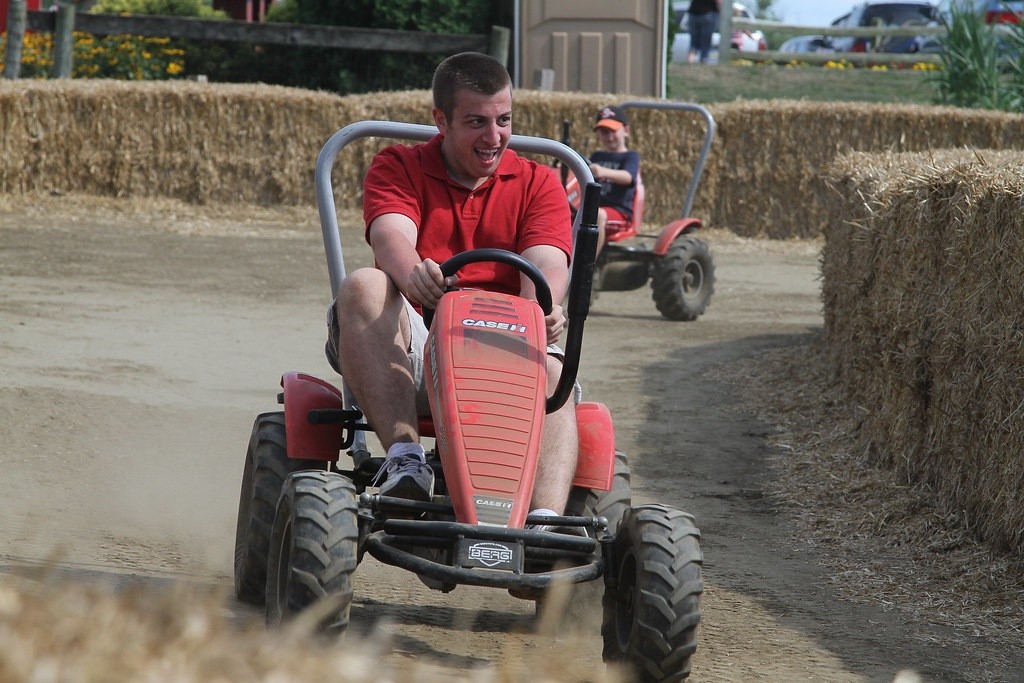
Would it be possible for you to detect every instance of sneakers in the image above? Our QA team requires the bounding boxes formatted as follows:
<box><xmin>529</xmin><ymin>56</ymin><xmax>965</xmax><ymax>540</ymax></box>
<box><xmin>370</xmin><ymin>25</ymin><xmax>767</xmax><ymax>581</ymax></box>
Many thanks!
<box><xmin>371</xmin><ymin>453</ymin><xmax>435</xmax><ymax>518</ymax></box>
<box><xmin>508</xmin><ymin>513</ymin><xmax>588</xmax><ymax>600</ymax></box>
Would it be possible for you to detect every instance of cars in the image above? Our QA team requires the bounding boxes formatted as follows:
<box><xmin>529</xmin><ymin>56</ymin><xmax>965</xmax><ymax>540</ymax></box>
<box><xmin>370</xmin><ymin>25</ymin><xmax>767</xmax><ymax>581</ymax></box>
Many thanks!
<box><xmin>671</xmin><ymin>2</ymin><xmax>768</xmax><ymax>65</ymax></box>
<box><xmin>936</xmin><ymin>1</ymin><xmax>1024</xmax><ymax>45</ymax></box>
<box><xmin>776</xmin><ymin>34</ymin><xmax>829</xmax><ymax>66</ymax></box>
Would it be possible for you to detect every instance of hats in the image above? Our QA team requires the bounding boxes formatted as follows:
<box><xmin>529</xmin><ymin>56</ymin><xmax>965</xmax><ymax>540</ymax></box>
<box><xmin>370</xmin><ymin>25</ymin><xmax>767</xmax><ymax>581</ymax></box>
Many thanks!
<box><xmin>593</xmin><ymin>105</ymin><xmax>627</xmax><ymax>132</ymax></box>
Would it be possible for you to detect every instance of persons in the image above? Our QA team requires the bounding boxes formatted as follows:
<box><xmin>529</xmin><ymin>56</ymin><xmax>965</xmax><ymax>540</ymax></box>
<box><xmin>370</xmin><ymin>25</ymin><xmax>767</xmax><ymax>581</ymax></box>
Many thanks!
<box><xmin>336</xmin><ymin>53</ymin><xmax>581</xmax><ymax>597</ymax></box>
<box><xmin>589</xmin><ymin>105</ymin><xmax>640</xmax><ymax>286</ymax></box>
<box><xmin>687</xmin><ymin>0</ymin><xmax>724</xmax><ymax>64</ymax></box>
<box><xmin>729</xmin><ymin>0</ymin><xmax>759</xmax><ymax>52</ymax></box>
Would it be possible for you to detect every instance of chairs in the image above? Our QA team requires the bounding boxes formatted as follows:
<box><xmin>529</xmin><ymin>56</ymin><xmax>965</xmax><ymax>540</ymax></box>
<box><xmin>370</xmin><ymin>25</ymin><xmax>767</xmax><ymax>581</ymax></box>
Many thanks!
<box><xmin>605</xmin><ymin>168</ymin><xmax>644</xmax><ymax>243</ymax></box>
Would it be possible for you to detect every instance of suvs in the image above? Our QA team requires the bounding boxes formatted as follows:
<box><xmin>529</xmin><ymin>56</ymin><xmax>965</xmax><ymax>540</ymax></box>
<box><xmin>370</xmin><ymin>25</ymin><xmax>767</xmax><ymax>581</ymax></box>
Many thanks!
<box><xmin>811</xmin><ymin>0</ymin><xmax>952</xmax><ymax>67</ymax></box>
